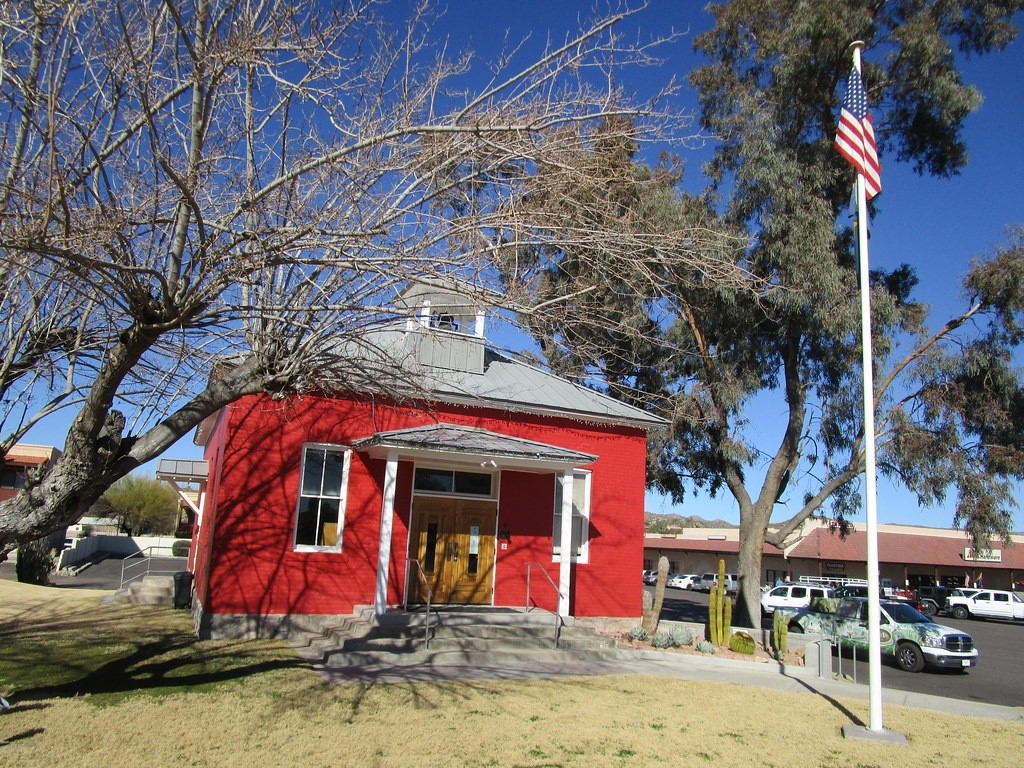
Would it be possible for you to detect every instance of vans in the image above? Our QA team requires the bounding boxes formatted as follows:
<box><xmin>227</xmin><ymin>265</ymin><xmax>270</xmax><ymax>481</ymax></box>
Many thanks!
<box><xmin>701</xmin><ymin>573</ymin><xmax>719</xmax><ymax>590</ymax></box>
<box><xmin>725</xmin><ymin>574</ymin><xmax>740</xmax><ymax>594</ymax></box>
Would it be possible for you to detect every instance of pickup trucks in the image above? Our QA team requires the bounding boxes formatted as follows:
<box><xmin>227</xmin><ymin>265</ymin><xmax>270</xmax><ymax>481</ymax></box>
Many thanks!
<box><xmin>831</xmin><ymin>586</ymin><xmax>922</xmax><ymax>614</ymax></box>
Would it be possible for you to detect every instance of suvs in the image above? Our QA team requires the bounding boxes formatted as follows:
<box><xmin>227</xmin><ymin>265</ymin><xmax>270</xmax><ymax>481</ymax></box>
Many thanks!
<box><xmin>955</xmin><ymin>587</ymin><xmax>1024</xmax><ymax>604</ymax></box>
<box><xmin>913</xmin><ymin>586</ymin><xmax>969</xmax><ymax>616</ymax></box>
<box><xmin>760</xmin><ymin>584</ymin><xmax>835</xmax><ymax>618</ymax></box>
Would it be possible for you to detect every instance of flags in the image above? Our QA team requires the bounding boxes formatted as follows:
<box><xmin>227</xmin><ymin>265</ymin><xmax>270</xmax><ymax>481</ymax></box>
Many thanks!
<box><xmin>834</xmin><ymin>65</ymin><xmax>882</xmax><ymax>200</ymax></box>
<box><xmin>964</xmin><ymin>574</ymin><xmax>970</xmax><ymax>587</ymax></box>
<box><xmin>979</xmin><ymin>576</ymin><xmax>983</xmax><ymax>588</ymax></box>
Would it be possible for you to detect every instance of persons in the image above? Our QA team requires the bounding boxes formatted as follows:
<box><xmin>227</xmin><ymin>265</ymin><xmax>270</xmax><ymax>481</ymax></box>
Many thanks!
<box><xmin>776</xmin><ymin>578</ymin><xmax>783</xmax><ymax>587</ymax></box>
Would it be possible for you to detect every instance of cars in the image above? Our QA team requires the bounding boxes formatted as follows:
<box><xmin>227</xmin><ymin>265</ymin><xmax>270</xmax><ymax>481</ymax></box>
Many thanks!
<box><xmin>788</xmin><ymin>595</ymin><xmax>978</xmax><ymax>674</ymax></box>
<box><xmin>643</xmin><ymin>570</ymin><xmax>676</xmax><ymax>586</ymax></box>
<box><xmin>666</xmin><ymin>575</ymin><xmax>677</xmax><ymax>587</ymax></box>
<box><xmin>691</xmin><ymin>576</ymin><xmax>703</xmax><ymax>591</ymax></box>
<box><xmin>671</xmin><ymin>575</ymin><xmax>699</xmax><ymax>591</ymax></box>
<box><xmin>944</xmin><ymin>589</ymin><xmax>1024</xmax><ymax>621</ymax></box>
